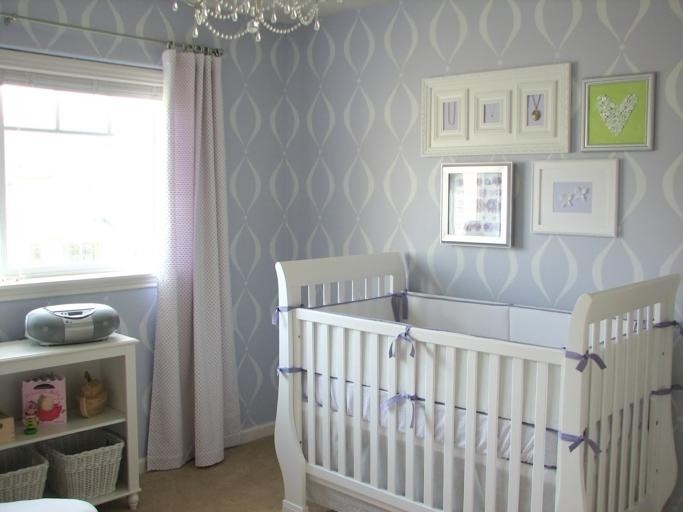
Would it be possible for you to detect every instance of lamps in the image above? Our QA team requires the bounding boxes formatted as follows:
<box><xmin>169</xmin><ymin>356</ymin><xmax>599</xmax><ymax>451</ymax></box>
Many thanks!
<box><xmin>172</xmin><ymin>0</ymin><xmax>322</xmax><ymax>43</ymax></box>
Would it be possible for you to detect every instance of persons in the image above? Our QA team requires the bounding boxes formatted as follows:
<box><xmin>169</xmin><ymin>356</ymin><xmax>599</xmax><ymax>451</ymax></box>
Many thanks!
<box><xmin>24</xmin><ymin>400</ymin><xmax>41</xmax><ymax>434</ymax></box>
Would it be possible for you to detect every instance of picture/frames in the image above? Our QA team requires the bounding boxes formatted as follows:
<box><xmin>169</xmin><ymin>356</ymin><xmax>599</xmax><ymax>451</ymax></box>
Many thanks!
<box><xmin>439</xmin><ymin>161</ymin><xmax>514</xmax><ymax>249</ymax></box>
<box><xmin>530</xmin><ymin>158</ymin><xmax>620</xmax><ymax>238</ymax></box>
<box><xmin>579</xmin><ymin>71</ymin><xmax>656</xmax><ymax>153</ymax></box>
<box><xmin>421</xmin><ymin>63</ymin><xmax>571</xmax><ymax>158</ymax></box>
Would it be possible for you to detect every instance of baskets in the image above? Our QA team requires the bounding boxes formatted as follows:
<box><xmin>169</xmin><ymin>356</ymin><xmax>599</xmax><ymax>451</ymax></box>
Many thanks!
<box><xmin>36</xmin><ymin>429</ymin><xmax>125</xmax><ymax>500</ymax></box>
<box><xmin>0</xmin><ymin>445</ymin><xmax>50</xmax><ymax>503</ymax></box>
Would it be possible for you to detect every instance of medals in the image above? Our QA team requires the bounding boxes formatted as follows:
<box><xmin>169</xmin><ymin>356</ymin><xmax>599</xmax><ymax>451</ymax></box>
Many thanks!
<box><xmin>530</xmin><ymin>109</ymin><xmax>542</xmax><ymax>123</ymax></box>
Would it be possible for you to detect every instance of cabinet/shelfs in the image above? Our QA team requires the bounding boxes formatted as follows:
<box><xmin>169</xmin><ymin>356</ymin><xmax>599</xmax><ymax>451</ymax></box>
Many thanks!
<box><xmin>0</xmin><ymin>332</ymin><xmax>144</xmax><ymax>511</ymax></box>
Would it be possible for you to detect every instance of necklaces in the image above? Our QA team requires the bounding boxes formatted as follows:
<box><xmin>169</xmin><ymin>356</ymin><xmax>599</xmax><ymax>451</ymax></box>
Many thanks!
<box><xmin>447</xmin><ymin>101</ymin><xmax>458</xmax><ymax>126</ymax></box>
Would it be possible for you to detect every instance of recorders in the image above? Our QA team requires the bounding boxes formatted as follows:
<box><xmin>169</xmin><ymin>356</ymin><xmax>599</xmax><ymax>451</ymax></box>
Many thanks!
<box><xmin>24</xmin><ymin>302</ymin><xmax>121</xmax><ymax>348</ymax></box>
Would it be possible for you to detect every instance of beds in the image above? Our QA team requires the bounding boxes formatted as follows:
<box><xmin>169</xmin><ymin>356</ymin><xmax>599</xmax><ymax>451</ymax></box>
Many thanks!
<box><xmin>273</xmin><ymin>250</ymin><xmax>683</xmax><ymax>512</ymax></box>
